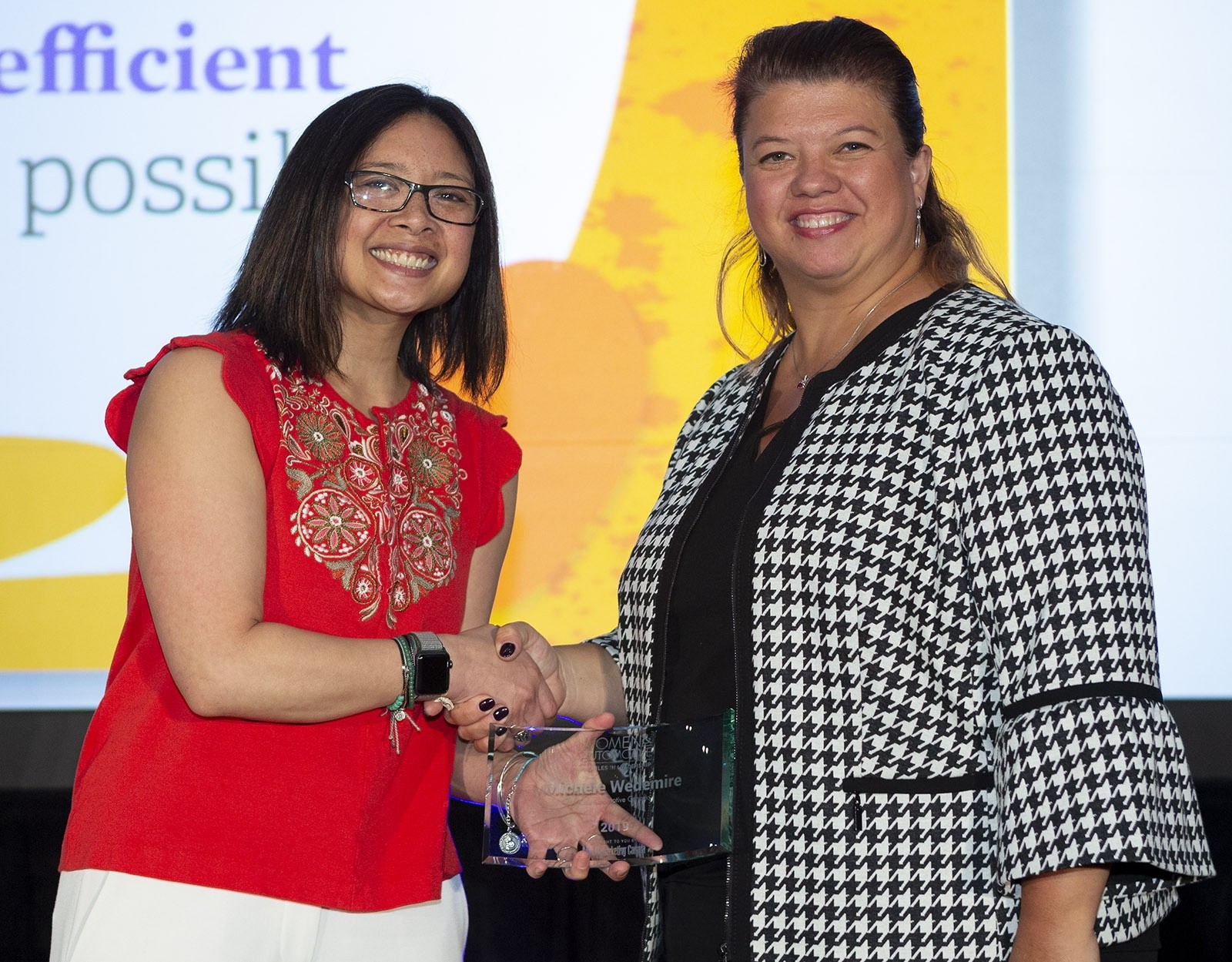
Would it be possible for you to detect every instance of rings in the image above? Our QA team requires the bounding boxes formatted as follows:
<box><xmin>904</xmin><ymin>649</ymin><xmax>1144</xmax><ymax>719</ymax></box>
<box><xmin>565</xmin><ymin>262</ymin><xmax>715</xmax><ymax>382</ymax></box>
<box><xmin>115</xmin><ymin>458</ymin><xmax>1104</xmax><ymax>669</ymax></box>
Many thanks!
<box><xmin>513</xmin><ymin>726</ymin><xmax>533</xmax><ymax>743</ymax></box>
<box><xmin>583</xmin><ymin>833</ymin><xmax>604</xmax><ymax>850</ymax></box>
<box><xmin>557</xmin><ymin>846</ymin><xmax>578</xmax><ymax>860</ymax></box>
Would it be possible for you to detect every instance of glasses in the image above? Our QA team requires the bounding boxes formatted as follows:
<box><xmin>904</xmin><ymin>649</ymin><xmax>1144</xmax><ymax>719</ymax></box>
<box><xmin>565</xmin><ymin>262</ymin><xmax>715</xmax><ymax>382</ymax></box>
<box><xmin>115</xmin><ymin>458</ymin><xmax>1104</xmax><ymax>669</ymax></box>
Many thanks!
<box><xmin>341</xmin><ymin>170</ymin><xmax>486</xmax><ymax>226</ymax></box>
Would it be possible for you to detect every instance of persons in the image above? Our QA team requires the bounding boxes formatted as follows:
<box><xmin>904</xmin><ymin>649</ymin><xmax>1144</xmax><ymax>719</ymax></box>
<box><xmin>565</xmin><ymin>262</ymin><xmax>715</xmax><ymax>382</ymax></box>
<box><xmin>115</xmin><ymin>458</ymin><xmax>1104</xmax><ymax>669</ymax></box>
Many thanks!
<box><xmin>423</xmin><ymin>15</ymin><xmax>1218</xmax><ymax>962</ymax></box>
<box><xmin>49</xmin><ymin>84</ymin><xmax>663</xmax><ymax>962</ymax></box>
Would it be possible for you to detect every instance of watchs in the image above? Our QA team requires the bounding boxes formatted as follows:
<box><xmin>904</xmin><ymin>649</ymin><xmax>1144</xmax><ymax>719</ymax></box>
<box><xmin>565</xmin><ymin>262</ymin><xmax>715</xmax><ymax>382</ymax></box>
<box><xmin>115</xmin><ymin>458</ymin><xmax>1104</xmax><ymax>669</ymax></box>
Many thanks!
<box><xmin>409</xmin><ymin>631</ymin><xmax>453</xmax><ymax>701</ymax></box>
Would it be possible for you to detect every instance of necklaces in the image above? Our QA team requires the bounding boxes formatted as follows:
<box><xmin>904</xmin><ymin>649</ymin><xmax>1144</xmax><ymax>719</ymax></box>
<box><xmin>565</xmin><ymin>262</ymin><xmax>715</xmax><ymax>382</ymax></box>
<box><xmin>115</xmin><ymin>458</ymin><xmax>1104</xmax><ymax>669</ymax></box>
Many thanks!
<box><xmin>792</xmin><ymin>262</ymin><xmax>928</xmax><ymax>388</ymax></box>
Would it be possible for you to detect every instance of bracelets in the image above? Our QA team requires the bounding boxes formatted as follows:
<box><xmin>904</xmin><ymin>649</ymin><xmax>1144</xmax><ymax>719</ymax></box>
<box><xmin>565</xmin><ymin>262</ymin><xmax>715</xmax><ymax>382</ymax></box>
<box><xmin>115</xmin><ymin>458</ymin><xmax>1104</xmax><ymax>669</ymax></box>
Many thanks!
<box><xmin>496</xmin><ymin>751</ymin><xmax>539</xmax><ymax>855</ymax></box>
<box><xmin>380</xmin><ymin>632</ymin><xmax>421</xmax><ymax>755</ymax></box>
